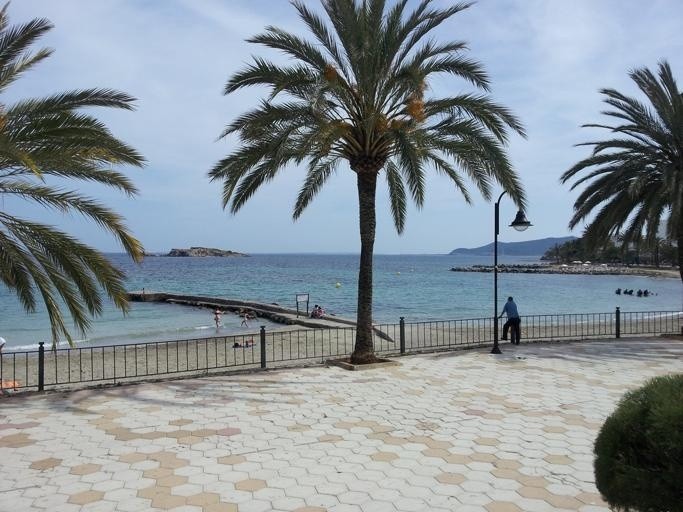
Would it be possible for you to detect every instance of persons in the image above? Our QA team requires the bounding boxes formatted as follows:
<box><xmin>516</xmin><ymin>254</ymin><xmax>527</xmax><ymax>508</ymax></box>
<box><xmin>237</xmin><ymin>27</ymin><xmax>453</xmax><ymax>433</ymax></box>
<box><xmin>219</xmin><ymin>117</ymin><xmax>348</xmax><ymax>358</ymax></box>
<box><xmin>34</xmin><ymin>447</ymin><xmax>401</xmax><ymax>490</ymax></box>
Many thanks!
<box><xmin>214</xmin><ymin>308</ymin><xmax>222</xmax><ymax>328</ymax></box>
<box><xmin>240</xmin><ymin>311</ymin><xmax>249</xmax><ymax>327</ymax></box>
<box><xmin>311</xmin><ymin>305</ymin><xmax>322</xmax><ymax>319</ymax></box>
<box><xmin>495</xmin><ymin>297</ymin><xmax>520</xmax><ymax>345</ymax></box>
<box><xmin>616</xmin><ymin>288</ymin><xmax>648</xmax><ymax>297</ymax></box>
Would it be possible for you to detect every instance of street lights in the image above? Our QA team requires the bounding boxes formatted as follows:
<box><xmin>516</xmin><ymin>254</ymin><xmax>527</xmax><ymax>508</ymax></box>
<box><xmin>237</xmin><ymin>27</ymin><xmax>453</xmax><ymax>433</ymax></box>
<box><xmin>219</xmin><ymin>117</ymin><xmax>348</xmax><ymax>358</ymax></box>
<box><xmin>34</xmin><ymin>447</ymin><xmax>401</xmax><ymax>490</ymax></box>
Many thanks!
<box><xmin>490</xmin><ymin>186</ymin><xmax>532</xmax><ymax>356</ymax></box>
